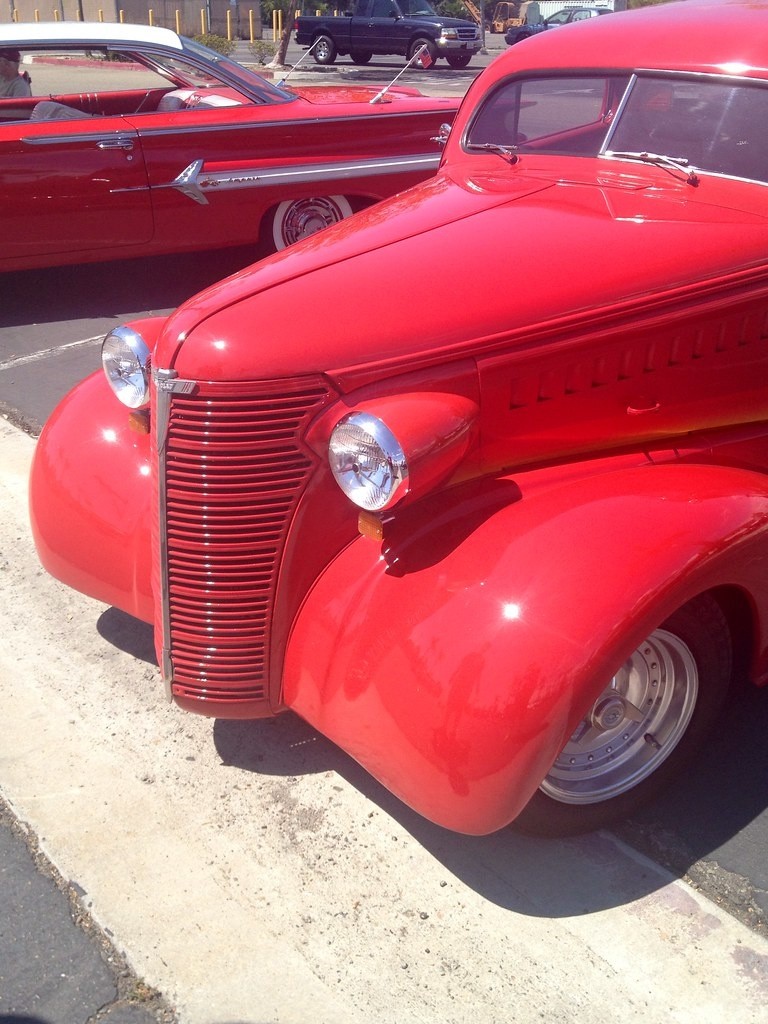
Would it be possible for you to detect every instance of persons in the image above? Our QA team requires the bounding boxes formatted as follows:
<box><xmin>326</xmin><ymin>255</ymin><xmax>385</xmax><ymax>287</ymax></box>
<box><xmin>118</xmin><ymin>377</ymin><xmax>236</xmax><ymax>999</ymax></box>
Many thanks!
<box><xmin>0</xmin><ymin>49</ymin><xmax>28</xmax><ymax>96</ymax></box>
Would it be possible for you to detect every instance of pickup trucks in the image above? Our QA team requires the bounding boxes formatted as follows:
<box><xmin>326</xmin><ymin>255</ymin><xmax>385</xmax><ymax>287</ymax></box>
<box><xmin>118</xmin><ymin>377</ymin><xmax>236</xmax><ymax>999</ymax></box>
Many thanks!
<box><xmin>292</xmin><ymin>1</ymin><xmax>484</xmax><ymax>70</ymax></box>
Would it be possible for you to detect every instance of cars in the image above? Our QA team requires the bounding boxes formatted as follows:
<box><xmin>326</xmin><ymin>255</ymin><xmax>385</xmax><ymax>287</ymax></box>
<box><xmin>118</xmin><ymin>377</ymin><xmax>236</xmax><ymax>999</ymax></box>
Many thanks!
<box><xmin>0</xmin><ymin>19</ymin><xmax>538</xmax><ymax>276</ymax></box>
<box><xmin>503</xmin><ymin>6</ymin><xmax>615</xmax><ymax>46</ymax></box>
<box><xmin>24</xmin><ymin>0</ymin><xmax>768</xmax><ymax>846</ymax></box>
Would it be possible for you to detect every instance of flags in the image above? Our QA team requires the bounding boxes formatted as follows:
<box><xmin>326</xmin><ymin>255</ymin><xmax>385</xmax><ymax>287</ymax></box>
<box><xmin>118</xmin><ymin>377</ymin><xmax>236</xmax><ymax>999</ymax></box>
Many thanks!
<box><xmin>420</xmin><ymin>49</ymin><xmax>433</xmax><ymax>68</ymax></box>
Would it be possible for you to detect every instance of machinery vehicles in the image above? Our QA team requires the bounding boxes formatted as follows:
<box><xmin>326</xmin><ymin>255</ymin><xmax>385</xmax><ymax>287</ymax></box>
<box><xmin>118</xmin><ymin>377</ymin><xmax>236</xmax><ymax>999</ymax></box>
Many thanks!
<box><xmin>462</xmin><ymin>0</ymin><xmax>528</xmax><ymax>34</ymax></box>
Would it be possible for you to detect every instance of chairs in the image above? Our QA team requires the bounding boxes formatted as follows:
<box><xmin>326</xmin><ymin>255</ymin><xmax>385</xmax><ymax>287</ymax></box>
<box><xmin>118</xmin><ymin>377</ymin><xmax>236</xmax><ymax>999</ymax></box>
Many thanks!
<box><xmin>30</xmin><ymin>101</ymin><xmax>92</xmax><ymax>119</ymax></box>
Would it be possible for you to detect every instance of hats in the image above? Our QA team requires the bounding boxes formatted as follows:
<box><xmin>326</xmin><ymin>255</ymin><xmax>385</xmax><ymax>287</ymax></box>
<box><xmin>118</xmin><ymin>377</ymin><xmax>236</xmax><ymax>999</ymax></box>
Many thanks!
<box><xmin>0</xmin><ymin>48</ymin><xmax>22</xmax><ymax>62</ymax></box>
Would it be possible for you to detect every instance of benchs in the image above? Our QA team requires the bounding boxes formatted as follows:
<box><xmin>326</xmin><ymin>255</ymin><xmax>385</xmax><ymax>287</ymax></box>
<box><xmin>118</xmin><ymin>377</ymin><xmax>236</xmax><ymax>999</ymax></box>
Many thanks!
<box><xmin>156</xmin><ymin>89</ymin><xmax>241</xmax><ymax>110</ymax></box>
